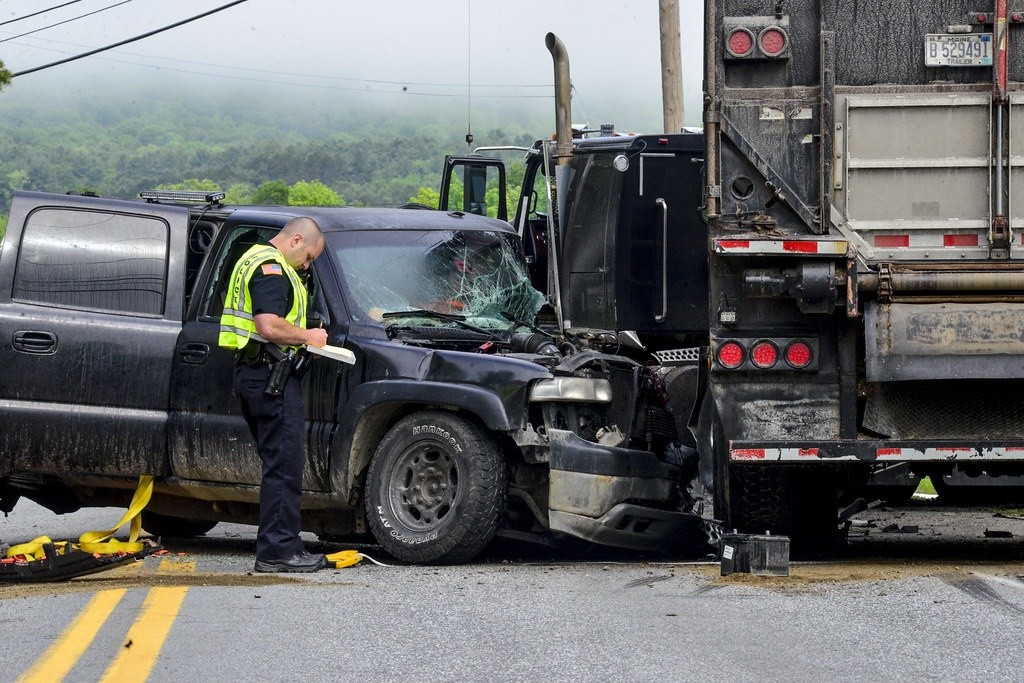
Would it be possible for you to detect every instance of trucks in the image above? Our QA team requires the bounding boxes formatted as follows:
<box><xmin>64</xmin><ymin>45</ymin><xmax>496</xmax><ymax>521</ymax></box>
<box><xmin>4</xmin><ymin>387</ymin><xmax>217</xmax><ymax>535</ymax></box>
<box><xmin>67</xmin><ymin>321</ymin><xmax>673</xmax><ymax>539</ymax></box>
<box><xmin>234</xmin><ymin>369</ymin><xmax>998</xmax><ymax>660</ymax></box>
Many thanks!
<box><xmin>423</xmin><ymin>0</ymin><xmax>1024</xmax><ymax>563</ymax></box>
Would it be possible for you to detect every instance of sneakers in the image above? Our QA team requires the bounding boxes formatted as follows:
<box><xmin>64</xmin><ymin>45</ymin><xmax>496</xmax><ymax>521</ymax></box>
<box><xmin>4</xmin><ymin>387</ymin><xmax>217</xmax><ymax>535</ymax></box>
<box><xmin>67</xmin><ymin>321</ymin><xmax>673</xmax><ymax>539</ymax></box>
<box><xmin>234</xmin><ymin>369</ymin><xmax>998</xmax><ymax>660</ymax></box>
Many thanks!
<box><xmin>253</xmin><ymin>554</ymin><xmax>322</xmax><ymax>572</ymax></box>
<box><xmin>299</xmin><ymin>549</ymin><xmax>328</xmax><ymax>571</ymax></box>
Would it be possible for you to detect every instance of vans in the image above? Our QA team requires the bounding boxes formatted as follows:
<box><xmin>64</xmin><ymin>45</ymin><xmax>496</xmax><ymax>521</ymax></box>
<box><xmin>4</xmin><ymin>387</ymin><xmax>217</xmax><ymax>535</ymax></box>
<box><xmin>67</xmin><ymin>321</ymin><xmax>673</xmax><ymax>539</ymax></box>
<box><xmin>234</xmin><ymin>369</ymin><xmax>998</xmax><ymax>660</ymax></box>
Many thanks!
<box><xmin>0</xmin><ymin>183</ymin><xmax>701</xmax><ymax>566</ymax></box>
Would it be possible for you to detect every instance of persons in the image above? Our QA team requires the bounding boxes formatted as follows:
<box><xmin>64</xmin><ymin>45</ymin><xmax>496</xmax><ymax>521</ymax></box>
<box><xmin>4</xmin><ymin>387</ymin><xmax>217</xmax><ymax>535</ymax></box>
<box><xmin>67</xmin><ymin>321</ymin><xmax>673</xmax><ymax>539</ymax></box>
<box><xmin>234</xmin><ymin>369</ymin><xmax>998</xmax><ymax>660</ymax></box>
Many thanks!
<box><xmin>218</xmin><ymin>216</ymin><xmax>328</xmax><ymax>574</ymax></box>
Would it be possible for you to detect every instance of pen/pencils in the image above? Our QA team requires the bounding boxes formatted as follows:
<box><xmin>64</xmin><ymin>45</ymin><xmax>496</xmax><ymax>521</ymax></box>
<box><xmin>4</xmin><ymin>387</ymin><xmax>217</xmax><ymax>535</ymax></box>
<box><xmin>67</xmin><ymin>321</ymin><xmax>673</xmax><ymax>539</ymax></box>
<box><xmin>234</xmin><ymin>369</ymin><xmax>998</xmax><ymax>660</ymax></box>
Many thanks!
<box><xmin>319</xmin><ymin>321</ymin><xmax>323</xmax><ymax>329</ymax></box>
